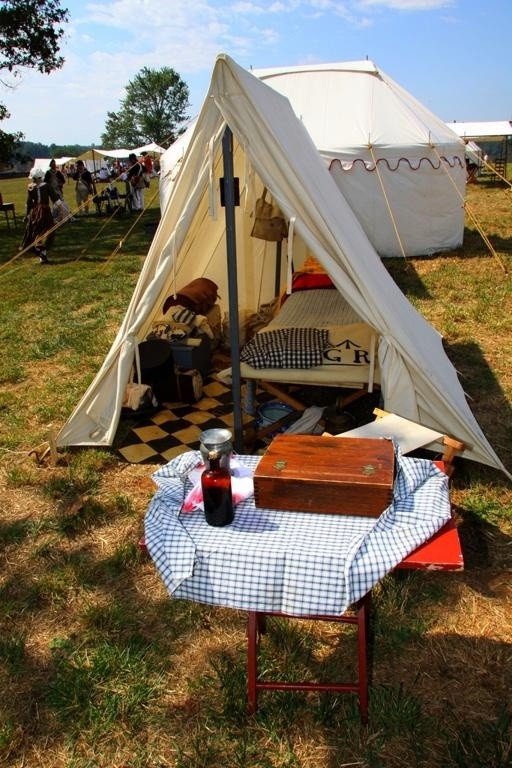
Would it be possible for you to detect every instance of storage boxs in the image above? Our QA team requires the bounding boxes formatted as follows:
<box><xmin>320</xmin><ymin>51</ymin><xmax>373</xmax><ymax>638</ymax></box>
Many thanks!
<box><xmin>132</xmin><ymin>329</ymin><xmax>212</xmax><ymax>403</ymax></box>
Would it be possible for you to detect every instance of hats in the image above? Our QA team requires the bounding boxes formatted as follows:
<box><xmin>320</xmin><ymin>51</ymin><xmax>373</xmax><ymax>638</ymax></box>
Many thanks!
<box><xmin>28</xmin><ymin>168</ymin><xmax>46</xmax><ymax>180</ymax></box>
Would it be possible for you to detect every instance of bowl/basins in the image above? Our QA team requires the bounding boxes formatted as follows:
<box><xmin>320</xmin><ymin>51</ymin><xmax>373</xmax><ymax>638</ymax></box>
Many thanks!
<box><xmin>256</xmin><ymin>401</ymin><xmax>295</xmax><ymax>437</ymax></box>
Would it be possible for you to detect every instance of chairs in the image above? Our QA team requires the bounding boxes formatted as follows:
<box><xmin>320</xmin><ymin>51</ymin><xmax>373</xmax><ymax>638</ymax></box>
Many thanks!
<box><xmin>490</xmin><ymin>158</ymin><xmax>505</xmax><ymax>185</ymax></box>
<box><xmin>0</xmin><ymin>194</ymin><xmax>18</xmax><ymax>229</ymax></box>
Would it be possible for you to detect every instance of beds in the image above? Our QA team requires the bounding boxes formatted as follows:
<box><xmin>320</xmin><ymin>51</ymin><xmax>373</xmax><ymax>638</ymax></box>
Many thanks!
<box><xmin>215</xmin><ymin>254</ymin><xmax>387</xmax><ymax>445</ymax></box>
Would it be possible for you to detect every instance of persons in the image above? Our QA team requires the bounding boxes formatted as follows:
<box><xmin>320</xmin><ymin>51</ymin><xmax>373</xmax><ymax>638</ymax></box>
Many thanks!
<box><xmin>72</xmin><ymin>160</ymin><xmax>93</xmax><ymax>216</ymax></box>
<box><xmin>140</xmin><ymin>151</ymin><xmax>152</xmax><ymax>188</ymax></box>
<box><xmin>32</xmin><ymin>141</ymin><xmax>169</xmax><ymax>184</ymax></box>
<box><xmin>23</xmin><ymin>167</ymin><xmax>70</xmax><ymax>263</ymax></box>
<box><xmin>43</xmin><ymin>158</ymin><xmax>65</xmax><ymax>204</ymax></box>
<box><xmin>127</xmin><ymin>153</ymin><xmax>147</xmax><ymax>211</ymax></box>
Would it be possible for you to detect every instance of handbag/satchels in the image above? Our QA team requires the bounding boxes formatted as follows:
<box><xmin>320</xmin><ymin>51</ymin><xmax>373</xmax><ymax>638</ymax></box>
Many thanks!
<box><xmin>249</xmin><ymin>197</ymin><xmax>289</xmax><ymax>243</ymax></box>
<box><xmin>130</xmin><ymin>175</ymin><xmax>145</xmax><ymax>190</ymax></box>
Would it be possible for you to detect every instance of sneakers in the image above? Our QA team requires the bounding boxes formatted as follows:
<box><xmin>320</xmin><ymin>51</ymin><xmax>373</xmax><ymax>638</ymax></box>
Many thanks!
<box><xmin>38</xmin><ymin>252</ymin><xmax>49</xmax><ymax>263</ymax></box>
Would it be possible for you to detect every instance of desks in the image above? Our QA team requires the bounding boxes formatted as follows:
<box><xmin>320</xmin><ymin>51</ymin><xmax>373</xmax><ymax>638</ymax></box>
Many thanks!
<box><xmin>92</xmin><ymin>189</ymin><xmax>135</xmax><ymax>214</ymax></box>
<box><xmin>133</xmin><ymin>448</ymin><xmax>467</xmax><ymax>729</ymax></box>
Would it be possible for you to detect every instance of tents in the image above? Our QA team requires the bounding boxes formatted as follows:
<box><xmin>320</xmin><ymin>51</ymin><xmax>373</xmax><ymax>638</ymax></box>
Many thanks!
<box><xmin>157</xmin><ymin>61</ymin><xmax>470</xmax><ymax>258</ymax></box>
<box><xmin>52</xmin><ymin>49</ymin><xmax>508</xmax><ymax>472</ymax></box>
<box><xmin>466</xmin><ymin>138</ymin><xmax>484</xmax><ymax>166</ymax></box>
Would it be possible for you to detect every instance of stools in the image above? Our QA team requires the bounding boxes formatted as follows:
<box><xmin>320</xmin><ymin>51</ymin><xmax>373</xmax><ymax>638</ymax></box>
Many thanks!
<box><xmin>324</xmin><ymin>409</ymin><xmax>466</xmax><ymax>484</ymax></box>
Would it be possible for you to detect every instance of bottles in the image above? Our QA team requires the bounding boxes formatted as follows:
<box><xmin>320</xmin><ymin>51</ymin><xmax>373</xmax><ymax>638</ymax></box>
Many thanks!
<box><xmin>202</xmin><ymin>451</ymin><xmax>234</xmax><ymax>527</ymax></box>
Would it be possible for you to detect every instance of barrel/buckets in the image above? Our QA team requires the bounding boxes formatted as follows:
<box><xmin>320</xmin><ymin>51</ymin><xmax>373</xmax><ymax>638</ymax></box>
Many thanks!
<box><xmin>256</xmin><ymin>401</ymin><xmax>295</xmax><ymax>444</ymax></box>
